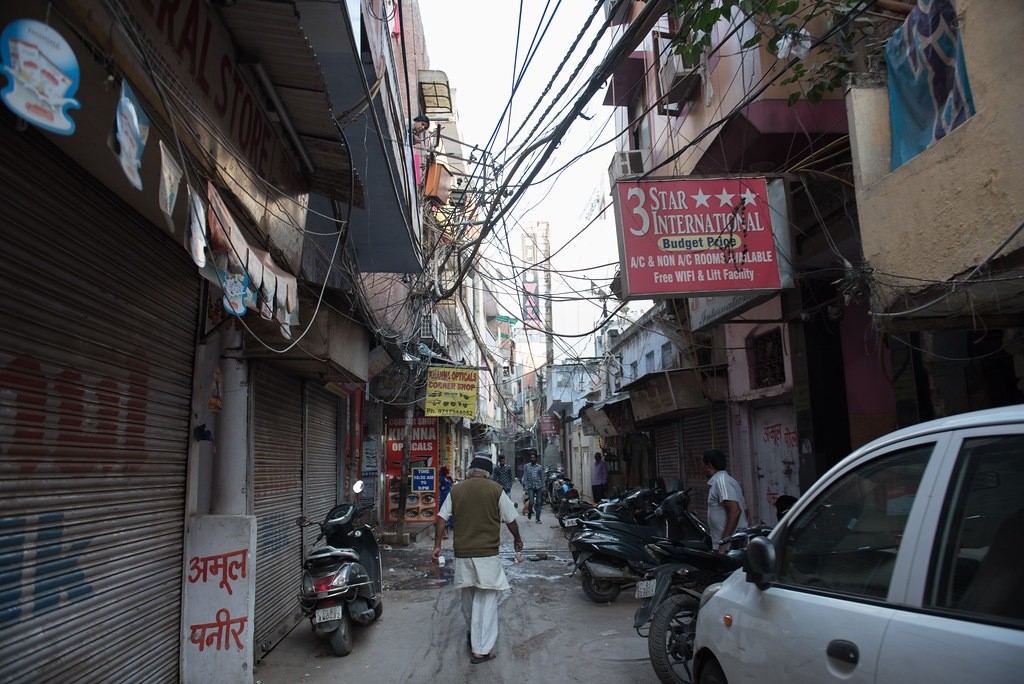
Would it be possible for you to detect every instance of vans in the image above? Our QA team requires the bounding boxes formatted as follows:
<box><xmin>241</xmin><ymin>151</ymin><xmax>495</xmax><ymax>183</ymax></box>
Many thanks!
<box><xmin>692</xmin><ymin>402</ymin><xmax>1024</xmax><ymax>684</ymax></box>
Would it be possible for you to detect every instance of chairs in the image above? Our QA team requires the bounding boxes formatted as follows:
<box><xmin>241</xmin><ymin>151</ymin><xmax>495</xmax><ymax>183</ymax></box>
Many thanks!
<box><xmin>955</xmin><ymin>508</ymin><xmax>1024</xmax><ymax>620</ymax></box>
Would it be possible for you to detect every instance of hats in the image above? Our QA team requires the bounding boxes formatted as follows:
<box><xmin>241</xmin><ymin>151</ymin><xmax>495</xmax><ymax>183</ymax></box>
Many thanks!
<box><xmin>470</xmin><ymin>456</ymin><xmax>493</xmax><ymax>474</ymax></box>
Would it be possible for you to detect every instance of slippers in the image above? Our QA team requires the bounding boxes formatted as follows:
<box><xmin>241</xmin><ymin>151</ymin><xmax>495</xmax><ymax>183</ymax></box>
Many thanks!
<box><xmin>470</xmin><ymin>650</ymin><xmax>496</xmax><ymax>664</ymax></box>
<box><xmin>466</xmin><ymin>630</ymin><xmax>471</xmax><ymax>645</ymax></box>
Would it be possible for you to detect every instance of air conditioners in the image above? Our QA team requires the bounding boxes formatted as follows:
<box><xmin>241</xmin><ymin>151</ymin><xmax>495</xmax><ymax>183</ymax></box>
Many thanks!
<box><xmin>609</xmin><ymin>148</ymin><xmax>652</xmax><ymax>192</ymax></box>
<box><xmin>421</xmin><ymin>314</ymin><xmax>448</xmax><ymax>348</ymax></box>
<box><xmin>658</xmin><ymin>46</ymin><xmax>700</xmax><ymax>105</ymax></box>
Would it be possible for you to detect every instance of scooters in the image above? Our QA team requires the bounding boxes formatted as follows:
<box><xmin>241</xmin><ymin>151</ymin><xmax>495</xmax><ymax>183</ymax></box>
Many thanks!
<box><xmin>544</xmin><ymin>470</ymin><xmax>585</xmax><ymax>526</ymax></box>
<box><xmin>565</xmin><ymin>484</ymin><xmax>714</xmax><ymax>603</ymax></box>
<box><xmin>295</xmin><ymin>480</ymin><xmax>383</xmax><ymax>656</ymax></box>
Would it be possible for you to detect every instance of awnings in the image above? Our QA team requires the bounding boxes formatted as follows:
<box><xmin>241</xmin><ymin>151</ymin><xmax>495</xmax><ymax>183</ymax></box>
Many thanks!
<box><xmin>611</xmin><ymin>361</ymin><xmax>727</xmax><ymax>393</ymax></box>
<box><xmin>607</xmin><ymin>98</ymin><xmax>848</xmax><ymax>301</ymax></box>
<box><xmin>562</xmin><ymin>391</ymin><xmax>630</xmax><ymax>422</ymax></box>
<box><xmin>215</xmin><ymin>0</ymin><xmax>365</xmax><ymax>206</ymax></box>
<box><xmin>418</xmin><ymin>70</ymin><xmax>453</xmax><ymax>113</ymax></box>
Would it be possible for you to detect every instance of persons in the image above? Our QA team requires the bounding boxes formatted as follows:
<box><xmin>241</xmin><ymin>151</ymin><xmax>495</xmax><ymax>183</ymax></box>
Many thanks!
<box><xmin>591</xmin><ymin>451</ymin><xmax>608</xmax><ymax>502</ymax></box>
<box><xmin>407</xmin><ymin>116</ymin><xmax>430</xmax><ymax>145</ymax></box>
<box><xmin>524</xmin><ymin>452</ymin><xmax>545</xmax><ymax>524</ymax></box>
<box><xmin>490</xmin><ymin>455</ymin><xmax>514</xmax><ymax>500</ymax></box>
<box><xmin>701</xmin><ymin>450</ymin><xmax>749</xmax><ymax>556</ymax></box>
<box><xmin>431</xmin><ymin>452</ymin><xmax>525</xmax><ymax>663</ymax></box>
<box><xmin>439</xmin><ymin>467</ymin><xmax>455</xmax><ymax>540</ymax></box>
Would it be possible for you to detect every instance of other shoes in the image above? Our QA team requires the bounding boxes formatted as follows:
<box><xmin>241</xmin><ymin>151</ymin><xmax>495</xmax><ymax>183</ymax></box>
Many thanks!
<box><xmin>528</xmin><ymin>512</ymin><xmax>531</xmax><ymax>519</ymax></box>
<box><xmin>535</xmin><ymin>519</ymin><xmax>542</xmax><ymax>523</ymax></box>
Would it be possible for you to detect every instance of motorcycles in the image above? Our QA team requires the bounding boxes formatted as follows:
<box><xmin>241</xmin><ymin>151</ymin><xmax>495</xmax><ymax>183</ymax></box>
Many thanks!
<box><xmin>633</xmin><ymin>494</ymin><xmax>800</xmax><ymax>684</ymax></box>
